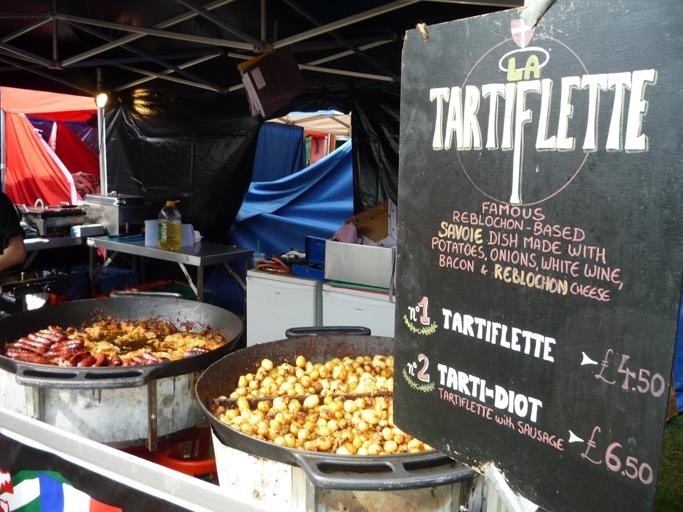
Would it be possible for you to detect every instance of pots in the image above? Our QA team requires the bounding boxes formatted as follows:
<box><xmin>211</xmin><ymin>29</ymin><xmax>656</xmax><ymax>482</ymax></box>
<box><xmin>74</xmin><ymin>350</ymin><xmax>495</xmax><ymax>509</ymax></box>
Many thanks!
<box><xmin>2</xmin><ymin>293</ymin><xmax>244</xmax><ymax>450</ymax></box>
<box><xmin>194</xmin><ymin>326</ymin><xmax>482</xmax><ymax>490</ymax></box>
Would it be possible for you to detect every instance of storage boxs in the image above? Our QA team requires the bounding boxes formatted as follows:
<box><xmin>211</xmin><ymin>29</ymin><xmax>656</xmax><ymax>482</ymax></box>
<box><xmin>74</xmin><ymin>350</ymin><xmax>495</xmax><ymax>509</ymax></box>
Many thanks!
<box><xmin>325</xmin><ymin>237</ymin><xmax>397</xmax><ymax>290</ymax></box>
<box><xmin>292</xmin><ymin>235</ymin><xmax>326</xmax><ymax>282</ymax></box>
<box><xmin>342</xmin><ymin>198</ymin><xmax>397</xmax><ymax>247</ymax></box>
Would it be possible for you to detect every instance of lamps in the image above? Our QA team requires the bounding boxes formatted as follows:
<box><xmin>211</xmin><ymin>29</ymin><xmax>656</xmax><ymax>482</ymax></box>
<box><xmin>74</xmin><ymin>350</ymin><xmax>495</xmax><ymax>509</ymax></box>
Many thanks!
<box><xmin>93</xmin><ymin>89</ymin><xmax>116</xmax><ymax>111</ymax></box>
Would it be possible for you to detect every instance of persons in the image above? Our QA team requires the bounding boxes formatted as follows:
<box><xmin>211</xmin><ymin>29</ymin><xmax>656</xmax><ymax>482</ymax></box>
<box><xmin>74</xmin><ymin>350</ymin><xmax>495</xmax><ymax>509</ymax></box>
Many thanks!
<box><xmin>1</xmin><ymin>191</ymin><xmax>28</xmax><ymax>272</ymax></box>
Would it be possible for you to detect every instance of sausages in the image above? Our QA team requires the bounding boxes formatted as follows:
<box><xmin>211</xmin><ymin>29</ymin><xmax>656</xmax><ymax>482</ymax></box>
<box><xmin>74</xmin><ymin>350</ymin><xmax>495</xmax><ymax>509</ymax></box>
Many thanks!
<box><xmin>5</xmin><ymin>324</ymin><xmax>212</xmax><ymax>367</ymax></box>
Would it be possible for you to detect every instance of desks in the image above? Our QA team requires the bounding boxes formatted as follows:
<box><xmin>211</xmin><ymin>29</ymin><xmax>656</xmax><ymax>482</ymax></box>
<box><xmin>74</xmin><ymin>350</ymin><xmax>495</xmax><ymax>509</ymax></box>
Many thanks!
<box><xmin>87</xmin><ymin>234</ymin><xmax>253</xmax><ymax>303</ymax></box>
